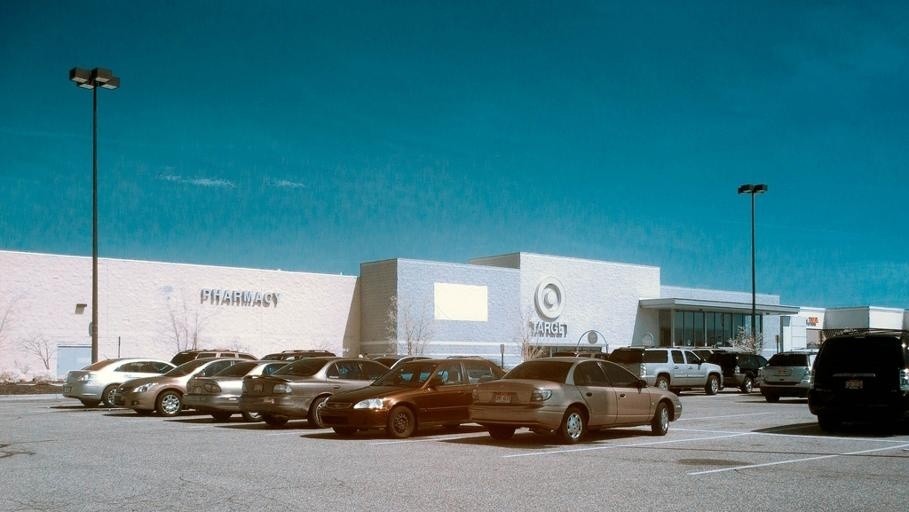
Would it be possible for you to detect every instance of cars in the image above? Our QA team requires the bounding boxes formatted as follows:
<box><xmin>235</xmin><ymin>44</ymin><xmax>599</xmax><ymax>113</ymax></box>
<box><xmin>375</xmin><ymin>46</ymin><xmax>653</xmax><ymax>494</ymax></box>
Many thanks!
<box><xmin>61</xmin><ymin>358</ymin><xmax>189</xmax><ymax>407</ymax></box>
<box><xmin>317</xmin><ymin>358</ymin><xmax>509</xmax><ymax>439</ymax></box>
<box><xmin>468</xmin><ymin>356</ymin><xmax>682</xmax><ymax>444</ymax></box>
<box><xmin>549</xmin><ymin>345</ymin><xmax>731</xmax><ymax>396</ymax></box>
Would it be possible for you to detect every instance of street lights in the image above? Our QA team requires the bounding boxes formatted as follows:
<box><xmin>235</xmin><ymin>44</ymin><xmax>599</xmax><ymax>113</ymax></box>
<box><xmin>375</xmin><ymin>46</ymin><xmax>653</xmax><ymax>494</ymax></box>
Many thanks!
<box><xmin>737</xmin><ymin>184</ymin><xmax>768</xmax><ymax>355</ymax></box>
<box><xmin>69</xmin><ymin>67</ymin><xmax>119</xmax><ymax>366</ymax></box>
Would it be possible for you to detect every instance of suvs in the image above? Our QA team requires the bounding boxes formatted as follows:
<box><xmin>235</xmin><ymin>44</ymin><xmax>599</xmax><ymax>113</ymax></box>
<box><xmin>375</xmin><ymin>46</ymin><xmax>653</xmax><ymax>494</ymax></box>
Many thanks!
<box><xmin>706</xmin><ymin>351</ymin><xmax>768</xmax><ymax>393</ymax></box>
<box><xmin>758</xmin><ymin>350</ymin><xmax>819</xmax><ymax>403</ymax></box>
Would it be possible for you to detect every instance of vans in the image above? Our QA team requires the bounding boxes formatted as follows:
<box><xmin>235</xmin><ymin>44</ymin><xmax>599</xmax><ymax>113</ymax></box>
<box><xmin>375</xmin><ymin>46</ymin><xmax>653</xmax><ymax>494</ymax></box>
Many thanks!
<box><xmin>808</xmin><ymin>329</ymin><xmax>909</xmax><ymax>434</ymax></box>
